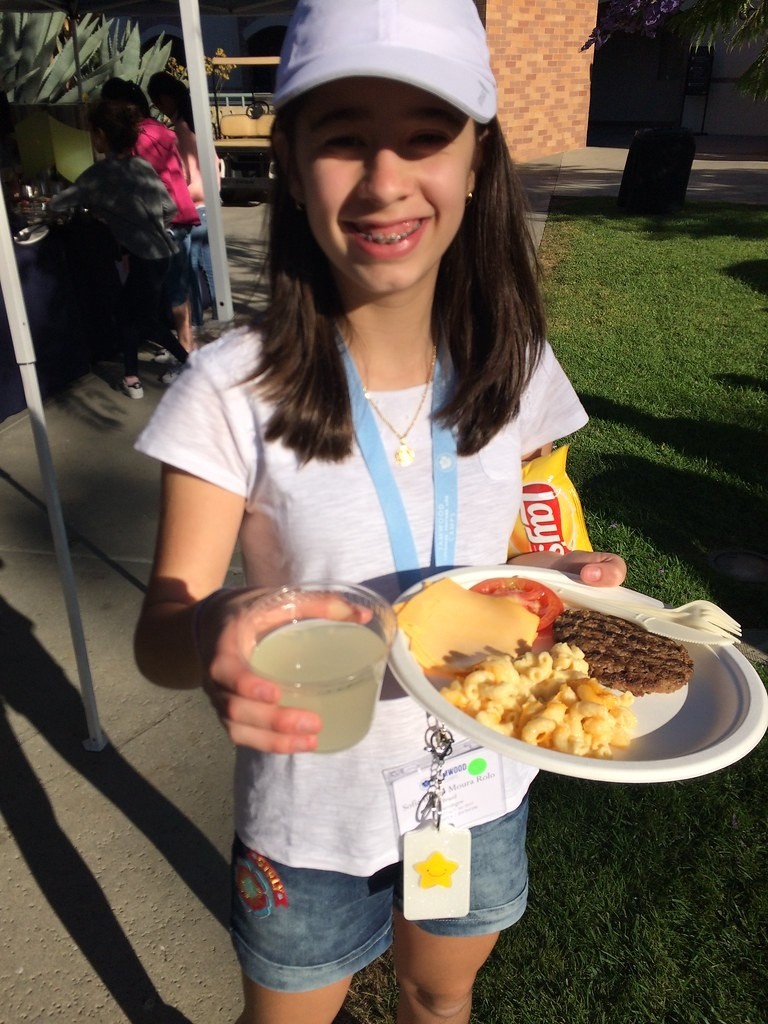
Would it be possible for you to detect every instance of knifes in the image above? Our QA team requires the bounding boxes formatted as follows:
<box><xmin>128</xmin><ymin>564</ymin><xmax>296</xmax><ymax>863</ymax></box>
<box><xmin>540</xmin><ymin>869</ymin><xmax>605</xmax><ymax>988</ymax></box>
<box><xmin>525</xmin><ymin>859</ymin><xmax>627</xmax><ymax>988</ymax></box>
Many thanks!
<box><xmin>520</xmin><ymin>576</ymin><xmax>736</xmax><ymax>646</ymax></box>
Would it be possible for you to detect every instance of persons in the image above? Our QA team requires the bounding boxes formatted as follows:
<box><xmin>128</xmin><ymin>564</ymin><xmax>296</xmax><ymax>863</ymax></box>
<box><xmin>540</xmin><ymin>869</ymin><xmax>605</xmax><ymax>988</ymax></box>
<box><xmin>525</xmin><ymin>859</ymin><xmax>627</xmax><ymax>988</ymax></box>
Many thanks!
<box><xmin>49</xmin><ymin>68</ymin><xmax>220</xmax><ymax>399</ymax></box>
<box><xmin>131</xmin><ymin>1</ymin><xmax>628</xmax><ymax>1023</ymax></box>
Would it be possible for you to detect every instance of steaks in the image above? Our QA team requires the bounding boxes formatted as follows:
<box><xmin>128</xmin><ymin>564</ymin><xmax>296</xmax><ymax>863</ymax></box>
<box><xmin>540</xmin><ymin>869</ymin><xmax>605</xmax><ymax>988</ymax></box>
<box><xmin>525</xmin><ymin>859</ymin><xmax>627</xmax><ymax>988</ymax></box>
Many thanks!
<box><xmin>551</xmin><ymin>608</ymin><xmax>694</xmax><ymax>698</ymax></box>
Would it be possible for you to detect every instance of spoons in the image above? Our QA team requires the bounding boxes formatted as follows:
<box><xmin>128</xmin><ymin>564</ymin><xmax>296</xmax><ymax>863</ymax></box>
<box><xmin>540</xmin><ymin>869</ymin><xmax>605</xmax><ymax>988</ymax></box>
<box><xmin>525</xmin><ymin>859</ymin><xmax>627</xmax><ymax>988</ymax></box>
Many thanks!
<box><xmin>17</xmin><ymin>223</ymin><xmax>47</xmax><ymax>240</ymax></box>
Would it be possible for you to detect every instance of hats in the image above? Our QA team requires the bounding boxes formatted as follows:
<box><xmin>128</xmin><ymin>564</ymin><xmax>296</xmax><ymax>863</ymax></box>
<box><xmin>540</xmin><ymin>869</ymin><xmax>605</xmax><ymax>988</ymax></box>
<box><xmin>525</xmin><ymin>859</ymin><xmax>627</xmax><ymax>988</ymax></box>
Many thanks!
<box><xmin>276</xmin><ymin>0</ymin><xmax>498</xmax><ymax>124</ymax></box>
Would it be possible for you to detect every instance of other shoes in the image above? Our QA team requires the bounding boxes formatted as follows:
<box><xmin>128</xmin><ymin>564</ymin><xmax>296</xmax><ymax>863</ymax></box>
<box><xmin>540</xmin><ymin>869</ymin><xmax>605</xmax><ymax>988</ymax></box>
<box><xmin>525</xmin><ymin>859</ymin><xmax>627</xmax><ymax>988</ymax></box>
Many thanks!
<box><xmin>122</xmin><ymin>378</ymin><xmax>143</xmax><ymax>398</ymax></box>
<box><xmin>162</xmin><ymin>362</ymin><xmax>188</xmax><ymax>384</ymax></box>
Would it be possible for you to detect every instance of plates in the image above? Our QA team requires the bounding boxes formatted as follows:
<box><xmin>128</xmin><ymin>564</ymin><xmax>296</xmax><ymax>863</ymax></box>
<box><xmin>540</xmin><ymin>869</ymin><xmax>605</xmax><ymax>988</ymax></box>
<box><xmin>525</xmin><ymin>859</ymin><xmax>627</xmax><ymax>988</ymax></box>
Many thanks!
<box><xmin>388</xmin><ymin>562</ymin><xmax>768</xmax><ymax>785</ymax></box>
<box><xmin>13</xmin><ymin>225</ymin><xmax>48</xmax><ymax>246</ymax></box>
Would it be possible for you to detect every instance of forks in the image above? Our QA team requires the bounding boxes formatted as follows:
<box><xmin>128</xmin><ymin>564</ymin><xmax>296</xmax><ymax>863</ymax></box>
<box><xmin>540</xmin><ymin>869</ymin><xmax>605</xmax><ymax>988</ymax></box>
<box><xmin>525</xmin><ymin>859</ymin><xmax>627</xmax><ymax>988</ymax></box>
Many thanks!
<box><xmin>516</xmin><ymin>575</ymin><xmax>743</xmax><ymax>644</ymax></box>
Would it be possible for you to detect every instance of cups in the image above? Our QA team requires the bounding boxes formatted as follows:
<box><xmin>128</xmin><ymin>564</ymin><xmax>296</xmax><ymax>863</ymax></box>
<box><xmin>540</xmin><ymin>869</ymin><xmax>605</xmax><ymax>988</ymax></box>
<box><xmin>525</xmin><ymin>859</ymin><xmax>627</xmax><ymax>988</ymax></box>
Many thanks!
<box><xmin>230</xmin><ymin>579</ymin><xmax>397</xmax><ymax>756</ymax></box>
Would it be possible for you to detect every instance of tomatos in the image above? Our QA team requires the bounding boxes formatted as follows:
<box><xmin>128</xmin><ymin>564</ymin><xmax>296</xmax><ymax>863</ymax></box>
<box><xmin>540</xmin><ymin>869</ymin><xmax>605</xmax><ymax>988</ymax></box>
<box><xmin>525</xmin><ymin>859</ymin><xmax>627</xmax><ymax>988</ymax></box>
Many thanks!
<box><xmin>467</xmin><ymin>577</ymin><xmax>562</xmax><ymax>631</ymax></box>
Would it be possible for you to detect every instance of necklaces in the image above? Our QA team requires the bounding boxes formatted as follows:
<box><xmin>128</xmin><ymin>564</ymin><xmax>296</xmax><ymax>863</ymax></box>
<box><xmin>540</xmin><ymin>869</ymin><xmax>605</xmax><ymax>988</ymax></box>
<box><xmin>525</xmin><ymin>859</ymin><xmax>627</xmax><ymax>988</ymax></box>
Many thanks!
<box><xmin>362</xmin><ymin>328</ymin><xmax>438</xmax><ymax>467</ymax></box>
